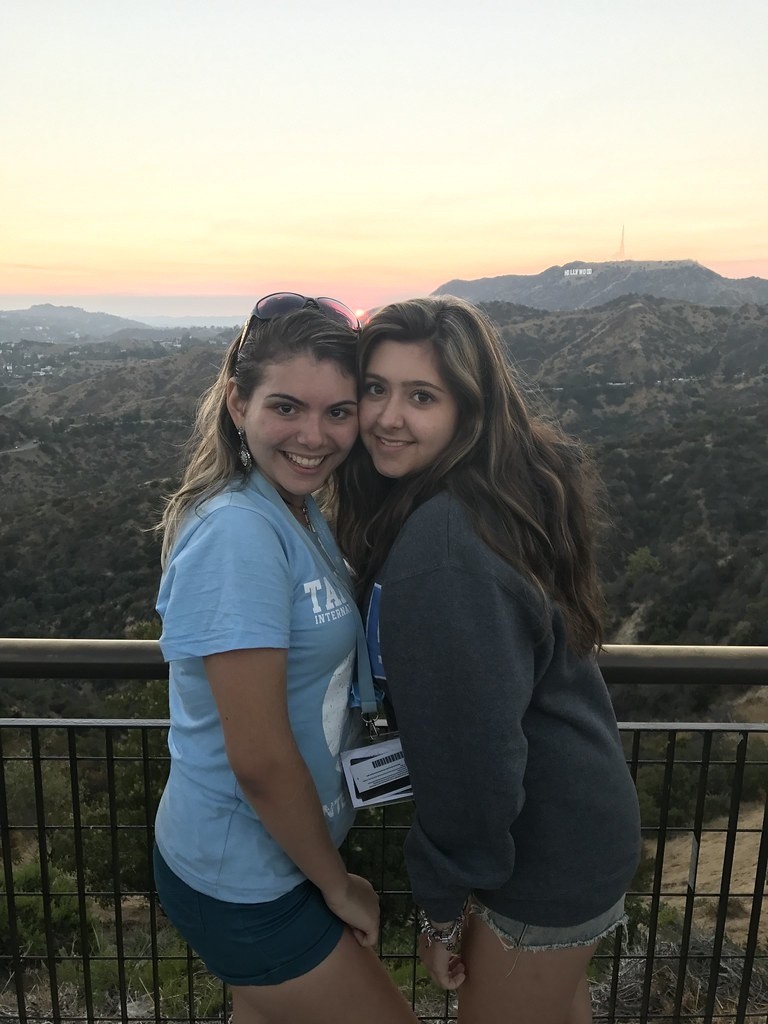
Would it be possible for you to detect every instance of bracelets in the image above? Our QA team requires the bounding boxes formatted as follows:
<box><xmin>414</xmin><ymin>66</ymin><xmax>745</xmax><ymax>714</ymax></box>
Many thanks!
<box><xmin>418</xmin><ymin>909</ymin><xmax>465</xmax><ymax>952</ymax></box>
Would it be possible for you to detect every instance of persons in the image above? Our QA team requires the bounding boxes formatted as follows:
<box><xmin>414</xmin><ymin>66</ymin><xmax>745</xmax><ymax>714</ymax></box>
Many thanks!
<box><xmin>152</xmin><ymin>292</ymin><xmax>421</xmax><ymax>1024</ymax></box>
<box><xmin>312</xmin><ymin>295</ymin><xmax>642</xmax><ymax>1024</ymax></box>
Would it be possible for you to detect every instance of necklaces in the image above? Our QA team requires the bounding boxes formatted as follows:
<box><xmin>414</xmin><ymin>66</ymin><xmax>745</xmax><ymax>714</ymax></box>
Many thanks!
<box><xmin>280</xmin><ymin>495</ymin><xmax>313</xmax><ymax>532</ymax></box>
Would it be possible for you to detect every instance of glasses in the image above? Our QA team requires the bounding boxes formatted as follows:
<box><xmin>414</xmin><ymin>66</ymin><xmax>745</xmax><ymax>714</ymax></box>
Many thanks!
<box><xmin>236</xmin><ymin>292</ymin><xmax>363</xmax><ymax>358</ymax></box>
